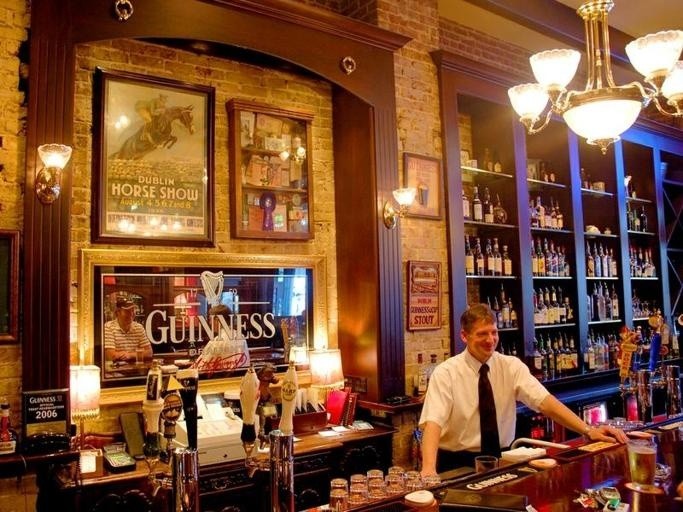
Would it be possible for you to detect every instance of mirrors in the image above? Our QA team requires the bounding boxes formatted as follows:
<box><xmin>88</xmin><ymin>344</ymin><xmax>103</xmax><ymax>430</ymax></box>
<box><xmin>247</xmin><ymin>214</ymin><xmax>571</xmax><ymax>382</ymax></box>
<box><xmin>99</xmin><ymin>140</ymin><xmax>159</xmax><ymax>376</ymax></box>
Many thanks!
<box><xmin>74</xmin><ymin>248</ymin><xmax>328</xmax><ymax>407</ymax></box>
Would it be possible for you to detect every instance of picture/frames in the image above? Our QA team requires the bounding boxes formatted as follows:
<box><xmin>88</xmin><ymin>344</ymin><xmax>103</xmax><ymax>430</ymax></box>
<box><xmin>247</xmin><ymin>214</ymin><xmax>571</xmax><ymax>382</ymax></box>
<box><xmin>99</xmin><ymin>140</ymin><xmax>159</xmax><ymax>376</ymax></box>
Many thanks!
<box><xmin>400</xmin><ymin>150</ymin><xmax>443</xmax><ymax>223</ymax></box>
<box><xmin>88</xmin><ymin>64</ymin><xmax>218</xmax><ymax>251</ymax></box>
<box><xmin>0</xmin><ymin>229</ymin><xmax>22</xmax><ymax>344</ymax></box>
<box><xmin>404</xmin><ymin>260</ymin><xmax>443</xmax><ymax>335</ymax></box>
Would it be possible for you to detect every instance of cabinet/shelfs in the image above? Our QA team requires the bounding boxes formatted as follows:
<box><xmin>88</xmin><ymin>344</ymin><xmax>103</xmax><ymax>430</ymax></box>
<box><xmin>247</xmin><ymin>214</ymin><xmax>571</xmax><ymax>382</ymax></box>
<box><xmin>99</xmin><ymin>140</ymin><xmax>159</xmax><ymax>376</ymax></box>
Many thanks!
<box><xmin>574</xmin><ymin>124</ymin><xmax>631</xmax><ymax>368</ymax></box>
<box><xmin>36</xmin><ymin>420</ymin><xmax>399</xmax><ymax>511</ymax></box>
<box><xmin>447</xmin><ymin>87</ymin><xmax>525</xmax><ymax>368</ymax></box>
<box><xmin>518</xmin><ymin>119</ymin><xmax>583</xmax><ymax>387</ymax></box>
<box><xmin>224</xmin><ymin>97</ymin><xmax>315</xmax><ymax>243</ymax></box>
<box><xmin>619</xmin><ymin>136</ymin><xmax>669</xmax><ymax>369</ymax></box>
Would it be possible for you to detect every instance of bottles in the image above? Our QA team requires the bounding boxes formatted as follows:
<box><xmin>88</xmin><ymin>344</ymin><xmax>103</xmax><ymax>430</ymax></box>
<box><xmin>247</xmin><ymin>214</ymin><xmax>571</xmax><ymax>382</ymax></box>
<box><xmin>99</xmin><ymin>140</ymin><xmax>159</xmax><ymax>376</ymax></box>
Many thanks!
<box><xmin>498</xmin><ymin>326</ymin><xmax>651</xmax><ymax>383</ymax></box>
<box><xmin>481</xmin><ymin>146</ymin><xmax>636</xmax><ymax>198</ymax></box>
<box><xmin>464</xmin><ymin>232</ymin><xmax>656</xmax><ymax>278</ymax></box>
<box><xmin>0</xmin><ymin>402</ymin><xmax>20</xmax><ymax>453</ymax></box>
<box><xmin>413</xmin><ymin>349</ymin><xmax>450</xmax><ymax>396</ymax></box>
<box><xmin>187</xmin><ymin>341</ymin><xmax>196</xmax><ymax>357</ymax></box>
<box><xmin>531</xmin><ymin>416</ymin><xmax>545</xmax><ymax>442</ymax></box>
<box><xmin>460</xmin><ymin>186</ymin><xmax>649</xmax><ymax>235</ymax></box>
<box><xmin>485</xmin><ymin>282</ymin><xmax>653</xmax><ymax>329</ymax></box>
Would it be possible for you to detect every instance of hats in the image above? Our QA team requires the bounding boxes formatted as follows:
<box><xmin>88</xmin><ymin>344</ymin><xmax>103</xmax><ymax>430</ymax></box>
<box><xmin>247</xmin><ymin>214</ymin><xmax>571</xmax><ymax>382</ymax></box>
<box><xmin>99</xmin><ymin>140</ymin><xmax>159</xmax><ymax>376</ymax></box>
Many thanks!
<box><xmin>117</xmin><ymin>298</ymin><xmax>138</xmax><ymax>309</ymax></box>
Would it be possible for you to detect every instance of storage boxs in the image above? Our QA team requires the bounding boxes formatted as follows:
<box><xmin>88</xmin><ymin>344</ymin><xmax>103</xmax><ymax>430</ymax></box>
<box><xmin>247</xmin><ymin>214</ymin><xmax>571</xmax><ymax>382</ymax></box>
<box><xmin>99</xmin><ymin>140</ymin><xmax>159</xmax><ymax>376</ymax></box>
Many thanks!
<box><xmin>263</xmin><ymin>401</ymin><xmax>328</xmax><ymax>440</ymax></box>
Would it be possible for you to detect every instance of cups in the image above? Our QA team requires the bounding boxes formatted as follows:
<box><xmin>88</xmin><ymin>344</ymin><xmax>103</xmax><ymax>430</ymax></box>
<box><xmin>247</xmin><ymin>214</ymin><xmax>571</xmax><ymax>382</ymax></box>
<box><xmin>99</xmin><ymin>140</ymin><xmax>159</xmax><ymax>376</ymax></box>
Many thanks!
<box><xmin>475</xmin><ymin>456</ymin><xmax>498</xmax><ymax>474</ymax></box>
<box><xmin>581</xmin><ymin>416</ymin><xmax>642</xmax><ymax>442</ymax></box>
<box><xmin>626</xmin><ymin>440</ymin><xmax>657</xmax><ymax>491</ymax></box>
<box><xmin>327</xmin><ymin>467</ymin><xmax>439</xmax><ymax>511</ymax></box>
<box><xmin>135</xmin><ymin>347</ymin><xmax>145</xmax><ymax>364</ymax></box>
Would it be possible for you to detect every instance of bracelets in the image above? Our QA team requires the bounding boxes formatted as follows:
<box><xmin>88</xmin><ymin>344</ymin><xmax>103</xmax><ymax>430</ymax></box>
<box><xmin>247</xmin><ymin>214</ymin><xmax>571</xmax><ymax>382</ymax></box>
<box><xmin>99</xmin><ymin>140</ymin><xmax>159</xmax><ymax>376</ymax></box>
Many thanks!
<box><xmin>583</xmin><ymin>424</ymin><xmax>594</xmax><ymax>436</ymax></box>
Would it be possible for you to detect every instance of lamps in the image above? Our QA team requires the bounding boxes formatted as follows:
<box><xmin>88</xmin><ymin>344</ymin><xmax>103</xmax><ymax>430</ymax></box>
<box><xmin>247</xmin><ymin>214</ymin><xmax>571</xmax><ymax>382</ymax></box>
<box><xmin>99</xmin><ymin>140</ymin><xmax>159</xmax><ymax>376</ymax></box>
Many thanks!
<box><xmin>380</xmin><ymin>184</ymin><xmax>418</xmax><ymax>231</ymax></box>
<box><xmin>309</xmin><ymin>346</ymin><xmax>348</xmax><ymax>428</ymax></box>
<box><xmin>505</xmin><ymin>2</ymin><xmax>683</xmax><ymax>156</ymax></box>
<box><xmin>67</xmin><ymin>362</ymin><xmax>102</xmax><ymax>452</ymax></box>
<box><xmin>31</xmin><ymin>140</ymin><xmax>73</xmax><ymax>206</ymax></box>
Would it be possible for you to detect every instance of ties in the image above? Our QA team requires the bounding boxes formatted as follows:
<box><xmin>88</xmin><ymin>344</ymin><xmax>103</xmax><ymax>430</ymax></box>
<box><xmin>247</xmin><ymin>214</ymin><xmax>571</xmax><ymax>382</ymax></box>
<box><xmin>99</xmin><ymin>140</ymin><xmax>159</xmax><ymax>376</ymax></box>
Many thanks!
<box><xmin>479</xmin><ymin>365</ymin><xmax>502</xmax><ymax>468</ymax></box>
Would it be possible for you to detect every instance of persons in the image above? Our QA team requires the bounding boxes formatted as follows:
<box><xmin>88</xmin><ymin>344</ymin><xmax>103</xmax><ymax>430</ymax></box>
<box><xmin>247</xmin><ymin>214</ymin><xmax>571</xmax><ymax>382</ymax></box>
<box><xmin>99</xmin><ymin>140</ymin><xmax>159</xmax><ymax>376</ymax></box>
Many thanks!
<box><xmin>418</xmin><ymin>305</ymin><xmax>631</xmax><ymax>489</ymax></box>
<box><xmin>104</xmin><ymin>298</ymin><xmax>154</xmax><ymax>363</ymax></box>
<box><xmin>190</xmin><ymin>304</ymin><xmax>251</xmax><ymax>371</ymax></box>
